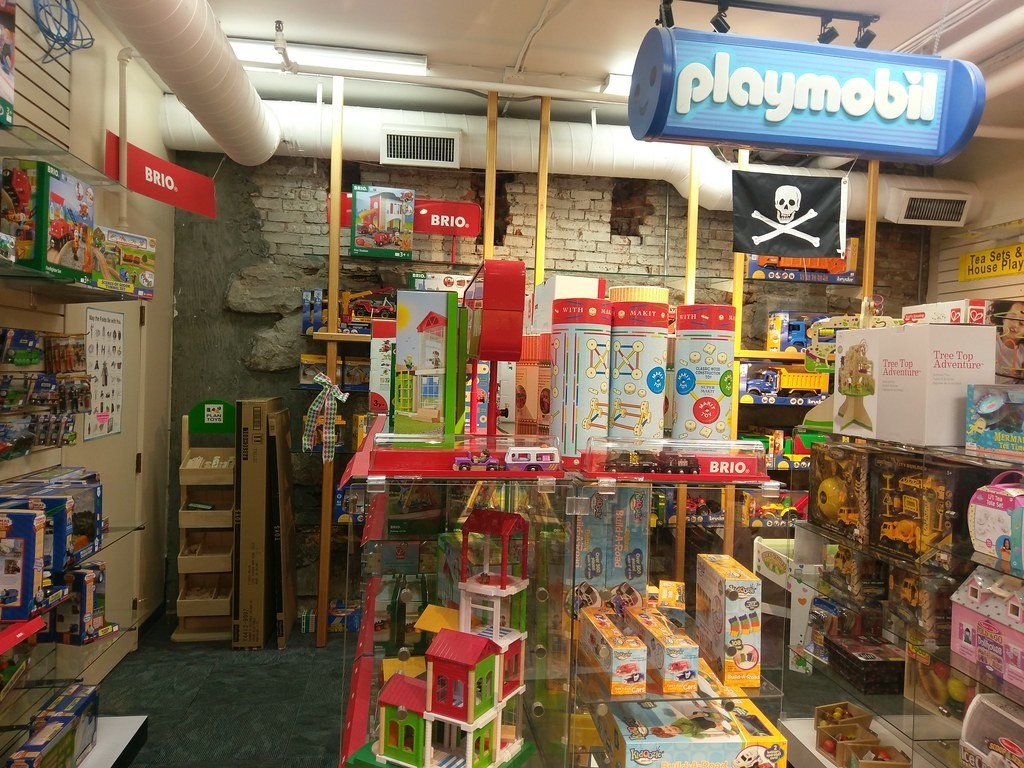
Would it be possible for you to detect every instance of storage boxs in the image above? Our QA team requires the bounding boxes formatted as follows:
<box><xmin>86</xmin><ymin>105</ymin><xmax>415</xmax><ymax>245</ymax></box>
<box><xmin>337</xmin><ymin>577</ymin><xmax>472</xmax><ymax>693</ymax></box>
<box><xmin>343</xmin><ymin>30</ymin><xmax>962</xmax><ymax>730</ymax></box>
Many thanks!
<box><xmin>0</xmin><ymin>157</ymin><xmax>158</xmax><ymax>298</ymax></box>
<box><xmin>6</xmin><ymin>685</ymin><xmax>100</xmax><ymax>768</ymax></box>
<box><xmin>0</xmin><ymin>466</ymin><xmax>119</xmax><ymax>648</ymax></box>
<box><xmin>437</xmin><ymin>274</ymin><xmax>1024</xmax><ymax>768</ymax></box>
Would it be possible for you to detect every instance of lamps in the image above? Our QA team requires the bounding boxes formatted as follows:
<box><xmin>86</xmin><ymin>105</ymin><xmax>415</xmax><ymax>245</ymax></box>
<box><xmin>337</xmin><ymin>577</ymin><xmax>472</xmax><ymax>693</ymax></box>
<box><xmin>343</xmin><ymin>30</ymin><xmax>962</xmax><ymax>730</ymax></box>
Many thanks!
<box><xmin>655</xmin><ymin>0</ymin><xmax>674</xmax><ymax>28</ymax></box>
<box><xmin>817</xmin><ymin>15</ymin><xmax>839</xmax><ymax>45</ymax></box>
<box><xmin>711</xmin><ymin>3</ymin><xmax>730</xmax><ymax>33</ymax></box>
<box><xmin>854</xmin><ymin>20</ymin><xmax>876</xmax><ymax>48</ymax></box>
<box><xmin>226</xmin><ymin>37</ymin><xmax>427</xmax><ymax>77</ymax></box>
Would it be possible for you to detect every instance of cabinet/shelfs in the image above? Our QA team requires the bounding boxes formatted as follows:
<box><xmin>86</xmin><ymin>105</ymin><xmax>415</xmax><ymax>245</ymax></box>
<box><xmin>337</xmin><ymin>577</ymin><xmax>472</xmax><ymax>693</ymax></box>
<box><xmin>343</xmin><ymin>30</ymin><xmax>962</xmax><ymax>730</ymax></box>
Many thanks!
<box><xmin>0</xmin><ymin>120</ymin><xmax>154</xmax><ymax>768</ymax></box>
<box><xmin>172</xmin><ymin>397</ymin><xmax>240</xmax><ymax>645</ymax></box>
<box><xmin>280</xmin><ymin>293</ymin><xmax>374</xmax><ymax>643</ymax></box>
<box><xmin>524</xmin><ymin>478</ymin><xmax>802</xmax><ymax>768</ymax></box>
<box><xmin>811</xmin><ymin>418</ymin><xmax>1024</xmax><ymax>768</ymax></box>
<box><xmin>703</xmin><ymin>236</ymin><xmax>873</xmax><ymax>589</ymax></box>
